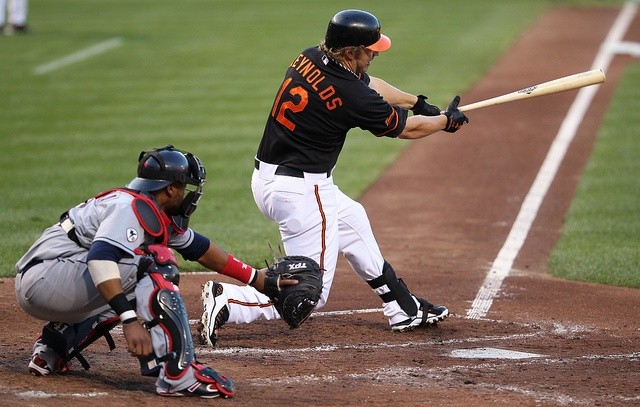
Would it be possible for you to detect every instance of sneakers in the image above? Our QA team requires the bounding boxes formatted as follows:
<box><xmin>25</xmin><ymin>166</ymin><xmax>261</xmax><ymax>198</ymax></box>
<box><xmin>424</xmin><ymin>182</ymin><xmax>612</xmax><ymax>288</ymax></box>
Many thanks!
<box><xmin>155</xmin><ymin>362</ymin><xmax>236</xmax><ymax>398</ymax></box>
<box><xmin>199</xmin><ymin>278</ymin><xmax>231</xmax><ymax>348</ymax></box>
<box><xmin>390</xmin><ymin>293</ymin><xmax>451</xmax><ymax>331</ymax></box>
<box><xmin>29</xmin><ymin>347</ymin><xmax>72</xmax><ymax>374</ymax></box>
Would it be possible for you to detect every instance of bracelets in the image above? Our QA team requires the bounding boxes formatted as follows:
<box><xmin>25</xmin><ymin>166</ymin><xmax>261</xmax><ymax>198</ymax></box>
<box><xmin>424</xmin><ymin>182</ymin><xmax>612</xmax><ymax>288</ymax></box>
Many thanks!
<box><xmin>107</xmin><ymin>291</ymin><xmax>139</xmax><ymax>325</ymax></box>
<box><xmin>220</xmin><ymin>255</ymin><xmax>256</xmax><ymax>284</ymax></box>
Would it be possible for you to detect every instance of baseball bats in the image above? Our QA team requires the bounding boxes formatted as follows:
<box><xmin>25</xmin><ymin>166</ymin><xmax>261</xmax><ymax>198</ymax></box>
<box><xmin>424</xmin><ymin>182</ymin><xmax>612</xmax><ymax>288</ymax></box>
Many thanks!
<box><xmin>439</xmin><ymin>68</ymin><xmax>606</xmax><ymax>115</ymax></box>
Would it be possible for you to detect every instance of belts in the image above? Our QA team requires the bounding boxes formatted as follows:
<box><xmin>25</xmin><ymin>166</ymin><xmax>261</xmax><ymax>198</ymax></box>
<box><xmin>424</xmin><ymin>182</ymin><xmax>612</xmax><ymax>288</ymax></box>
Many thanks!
<box><xmin>254</xmin><ymin>156</ymin><xmax>335</xmax><ymax>178</ymax></box>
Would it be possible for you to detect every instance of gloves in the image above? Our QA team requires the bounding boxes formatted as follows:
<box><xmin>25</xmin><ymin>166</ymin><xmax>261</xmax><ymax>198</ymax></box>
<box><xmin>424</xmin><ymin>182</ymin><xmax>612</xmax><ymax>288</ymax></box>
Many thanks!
<box><xmin>442</xmin><ymin>95</ymin><xmax>470</xmax><ymax>133</ymax></box>
<box><xmin>411</xmin><ymin>94</ymin><xmax>441</xmax><ymax>116</ymax></box>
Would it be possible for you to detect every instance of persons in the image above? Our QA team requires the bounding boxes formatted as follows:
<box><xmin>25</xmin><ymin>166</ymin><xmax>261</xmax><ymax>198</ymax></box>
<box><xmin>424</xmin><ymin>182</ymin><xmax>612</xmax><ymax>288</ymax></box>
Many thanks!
<box><xmin>196</xmin><ymin>11</ymin><xmax>466</xmax><ymax>348</ymax></box>
<box><xmin>14</xmin><ymin>145</ymin><xmax>300</xmax><ymax>397</ymax></box>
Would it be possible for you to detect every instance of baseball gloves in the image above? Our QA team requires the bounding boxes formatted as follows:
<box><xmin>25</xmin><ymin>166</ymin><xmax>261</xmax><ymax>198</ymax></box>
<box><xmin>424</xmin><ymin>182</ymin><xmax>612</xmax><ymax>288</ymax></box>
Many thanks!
<box><xmin>266</xmin><ymin>256</ymin><xmax>322</xmax><ymax>327</ymax></box>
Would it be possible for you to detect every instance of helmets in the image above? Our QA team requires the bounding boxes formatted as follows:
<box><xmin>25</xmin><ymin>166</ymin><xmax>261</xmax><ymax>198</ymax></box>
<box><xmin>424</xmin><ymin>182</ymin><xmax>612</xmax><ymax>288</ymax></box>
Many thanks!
<box><xmin>129</xmin><ymin>148</ymin><xmax>206</xmax><ymax>235</ymax></box>
<box><xmin>325</xmin><ymin>9</ymin><xmax>391</xmax><ymax>53</ymax></box>
